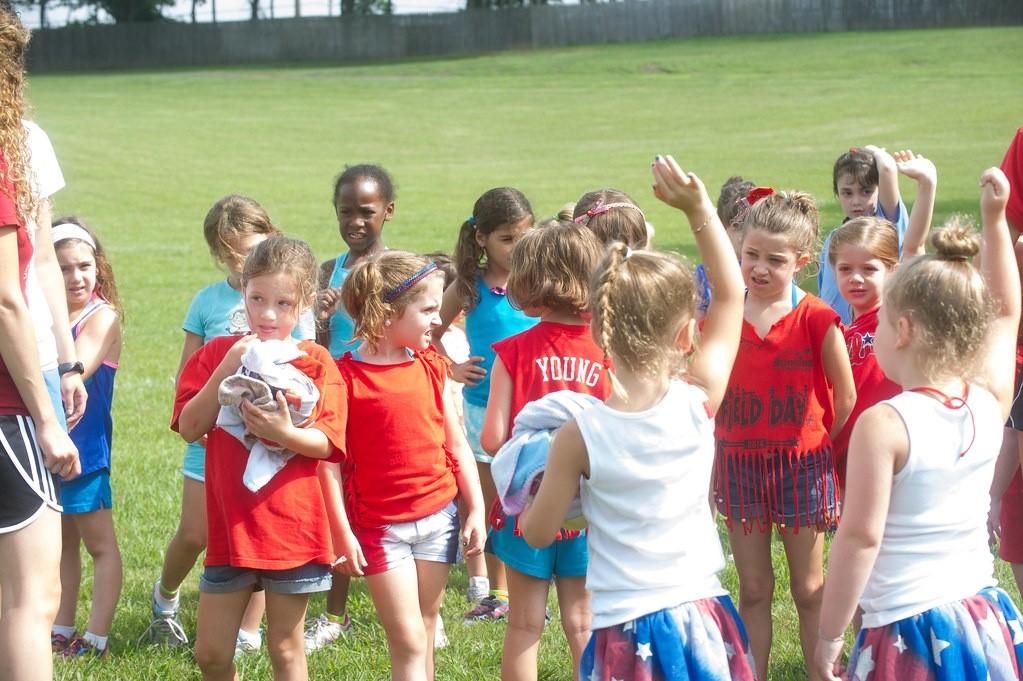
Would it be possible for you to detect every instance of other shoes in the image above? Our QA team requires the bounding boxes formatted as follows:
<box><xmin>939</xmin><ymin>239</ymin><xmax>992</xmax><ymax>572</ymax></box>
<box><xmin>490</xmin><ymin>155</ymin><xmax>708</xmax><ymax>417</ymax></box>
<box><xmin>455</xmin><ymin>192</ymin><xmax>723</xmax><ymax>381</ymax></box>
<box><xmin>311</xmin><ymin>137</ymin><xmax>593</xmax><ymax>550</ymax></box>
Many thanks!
<box><xmin>49</xmin><ymin>629</ymin><xmax>81</xmax><ymax>654</ymax></box>
<box><xmin>61</xmin><ymin>636</ymin><xmax>111</xmax><ymax>666</ymax></box>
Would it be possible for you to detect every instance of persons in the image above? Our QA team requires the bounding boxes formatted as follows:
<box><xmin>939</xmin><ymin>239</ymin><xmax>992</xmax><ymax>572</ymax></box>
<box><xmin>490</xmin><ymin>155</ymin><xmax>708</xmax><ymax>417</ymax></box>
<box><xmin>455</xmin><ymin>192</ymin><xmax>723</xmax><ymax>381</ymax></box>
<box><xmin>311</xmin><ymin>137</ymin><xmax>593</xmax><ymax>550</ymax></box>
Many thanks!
<box><xmin>50</xmin><ymin>218</ymin><xmax>127</xmax><ymax>658</ymax></box>
<box><xmin>813</xmin><ymin>125</ymin><xmax>1022</xmax><ymax>681</ymax></box>
<box><xmin>429</xmin><ymin>186</ymin><xmax>656</xmax><ymax>681</ymax></box>
<box><xmin>691</xmin><ymin>177</ymin><xmax>758</xmax><ymax>319</ymax></box>
<box><xmin>522</xmin><ymin>153</ymin><xmax>759</xmax><ymax>681</ymax></box>
<box><xmin>150</xmin><ymin>190</ymin><xmax>318</xmax><ymax>654</ymax></box>
<box><xmin>0</xmin><ymin>0</ymin><xmax>87</xmax><ymax>681</ymax></box>
<box><xmin>300</xmin><ymin>162</ymin><xmax>453</xmax><ymax>651</ymax></box>
<box><xmin>684</xmin><ymin>189</ymin><xmax>860</xmax><ymax>681</ymax></box>
<box><xmin>169</xmin><ymin>235</ymin><xmax>348</xmax><ymax>681</ymax></box>
<box><xmin>320</xmin><ymin>249</ymin><xmax>486</xmax><ymax>681</ymax></box>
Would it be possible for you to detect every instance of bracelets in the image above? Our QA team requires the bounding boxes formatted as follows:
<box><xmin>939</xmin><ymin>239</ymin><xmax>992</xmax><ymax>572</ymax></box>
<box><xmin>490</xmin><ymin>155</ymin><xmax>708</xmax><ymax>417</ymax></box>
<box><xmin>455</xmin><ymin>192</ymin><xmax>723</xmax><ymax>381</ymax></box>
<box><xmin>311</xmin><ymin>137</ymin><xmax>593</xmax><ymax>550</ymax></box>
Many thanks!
<box><xmin>816</xmin><ymin>630</ymin><xmax>845</xmax><ymax>642</ymax></box>
<box><xmin>690</xmin><ymin>208</ymin><xmax>718</xmax><ymax>232</ymax></box>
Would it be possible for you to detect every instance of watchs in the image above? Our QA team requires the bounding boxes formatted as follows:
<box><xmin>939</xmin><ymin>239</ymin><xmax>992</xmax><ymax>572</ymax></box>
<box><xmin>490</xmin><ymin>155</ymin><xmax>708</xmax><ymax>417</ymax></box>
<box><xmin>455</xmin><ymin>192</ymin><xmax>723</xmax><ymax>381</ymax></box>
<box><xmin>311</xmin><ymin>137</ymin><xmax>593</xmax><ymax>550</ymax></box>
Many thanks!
<box><xmin>57</xmin><ymin>361</ymin><xmax>84</xmax><ymax>375</ymax></box>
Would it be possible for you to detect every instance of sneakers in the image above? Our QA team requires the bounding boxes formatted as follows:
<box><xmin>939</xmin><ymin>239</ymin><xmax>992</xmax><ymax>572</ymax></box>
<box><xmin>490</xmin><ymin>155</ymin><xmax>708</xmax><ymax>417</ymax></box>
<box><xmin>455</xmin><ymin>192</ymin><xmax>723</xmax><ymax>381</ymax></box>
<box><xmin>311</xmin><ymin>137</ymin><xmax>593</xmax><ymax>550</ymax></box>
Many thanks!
<box><xmin>136</xmin><ymin>576</ymin><xmax>188</xmax><ymax>655</ymax></box>
<box><xmin>433</xmin><ymin>612</ymin><xmax>450</xmax><ymax>649</ymax></box>
<box><xmin>303</xmin><ymin>613</ymin><xmax>353</xmax><ymax>656</ymax></box>
<box><xmin>462</xmin><ymin>594</ymin><xmax>509</xmax><ymax>627</ymax></box>
<box><xmin>465</xmin><ymin>586</ymin><xmax>491</xmax><ymax>604</ymax></box>
<box><xmin>230</xmin><ymin>627</ymin><xmax>264</xmax><ymax>661</ymax></box>
